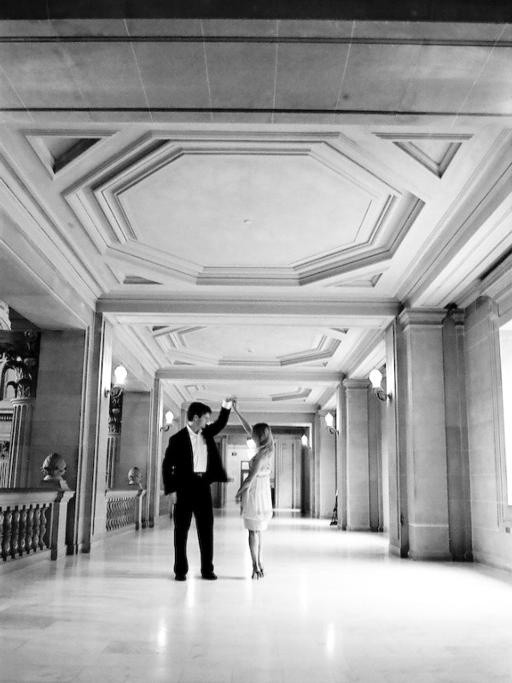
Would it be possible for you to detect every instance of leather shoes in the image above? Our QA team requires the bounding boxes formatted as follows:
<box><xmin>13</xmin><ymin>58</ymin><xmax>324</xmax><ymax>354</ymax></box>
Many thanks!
<box><xmin>175</xmin><ymin>571</ymin><xmax>216</xmax><ymax>582</ymax></box>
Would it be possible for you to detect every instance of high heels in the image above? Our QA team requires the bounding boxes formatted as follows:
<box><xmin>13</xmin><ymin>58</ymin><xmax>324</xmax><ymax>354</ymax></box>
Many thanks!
<box><xmin>252</xmin><ymin>568</ymin><xmax>264</xmax><ymax>579</ymax></box>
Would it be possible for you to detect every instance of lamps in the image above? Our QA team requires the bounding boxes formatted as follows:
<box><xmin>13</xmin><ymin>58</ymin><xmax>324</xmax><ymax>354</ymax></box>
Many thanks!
<box><xmin>324</xmin><ymin>412</ymin><xmax>339</xmax><ymax>435</ymax></box>
<box><xmin>368</xmin><ymin>367</ymin><xmax>393</xmax><ymax>402</ymax></box>
<box><xmin>160</xmin><ymin>410</ymin><xmax>174</xmax><ymax>432</ymax></box>
<box><xmin>104</xmin><ymin>363</ymin><xmax>127</xmax><ymax>398</ymax></box>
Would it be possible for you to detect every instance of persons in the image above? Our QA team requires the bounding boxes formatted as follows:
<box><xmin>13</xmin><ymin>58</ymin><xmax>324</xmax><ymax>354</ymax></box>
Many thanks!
<box><xmin>162</xmin><ymin>396</ymin><xmax>233</xmax><ymax>579</ymax></box>
<box><xmin>231</xmin><ymin>398</ymin><xmax>274</xmax><ymax>579</ymax></box>
